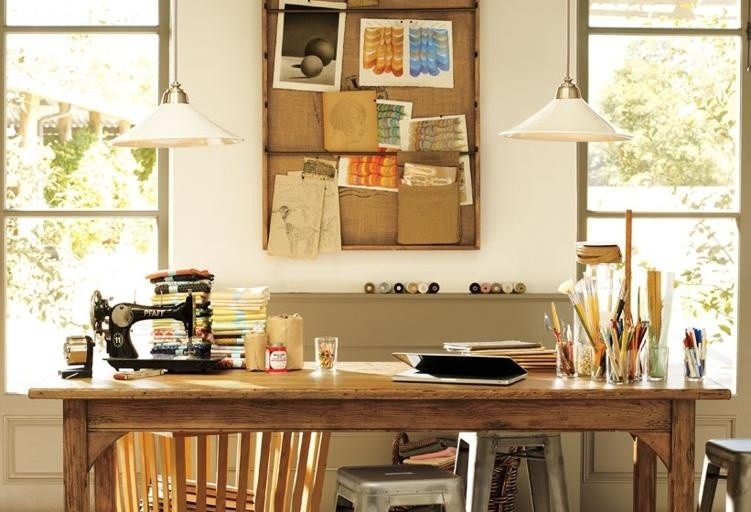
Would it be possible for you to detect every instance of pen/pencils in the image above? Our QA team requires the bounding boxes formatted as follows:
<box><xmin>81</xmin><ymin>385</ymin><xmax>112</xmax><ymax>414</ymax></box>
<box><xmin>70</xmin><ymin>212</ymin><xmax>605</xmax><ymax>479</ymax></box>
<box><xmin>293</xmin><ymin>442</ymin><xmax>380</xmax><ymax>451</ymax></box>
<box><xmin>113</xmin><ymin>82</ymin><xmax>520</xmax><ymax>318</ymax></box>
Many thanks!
<box><xmin>544</xmin><ymin>300</ymin><xmax>573</xmax><ymax>376</ymax></box>
<box><xmin>683</xmin><ymin>328</ymin><xmax>707</xmax><ymax>378</ymax></box>
<box><xmin>602</xmin><ymin>286</ymin><xmax>648</xmax><ymax>386</ymax></box>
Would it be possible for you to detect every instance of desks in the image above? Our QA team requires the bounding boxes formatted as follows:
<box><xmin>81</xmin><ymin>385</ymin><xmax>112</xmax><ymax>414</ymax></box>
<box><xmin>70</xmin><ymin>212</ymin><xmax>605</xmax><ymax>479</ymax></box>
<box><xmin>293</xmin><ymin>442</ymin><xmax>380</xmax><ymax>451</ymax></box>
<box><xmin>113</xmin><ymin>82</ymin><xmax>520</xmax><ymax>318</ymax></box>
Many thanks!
<box><xmin>27</xmin><ymin>358</ymin><xmax>734</xmax><ymax>512</ymax></box>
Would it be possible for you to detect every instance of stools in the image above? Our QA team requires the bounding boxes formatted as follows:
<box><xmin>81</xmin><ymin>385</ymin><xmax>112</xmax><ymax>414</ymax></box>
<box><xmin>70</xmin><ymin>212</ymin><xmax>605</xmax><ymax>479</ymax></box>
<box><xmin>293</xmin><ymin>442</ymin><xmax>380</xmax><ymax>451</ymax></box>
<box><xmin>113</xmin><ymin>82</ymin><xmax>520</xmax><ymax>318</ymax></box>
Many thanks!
<box><xmin>334</xmin><ymin>428</ymin><xmax>569</xmax><ymax>511</ymax></box>
<box><xmin>698</xmin><ymin>431</ymin><xmax>751</xmax><ymax>512</ymax></box>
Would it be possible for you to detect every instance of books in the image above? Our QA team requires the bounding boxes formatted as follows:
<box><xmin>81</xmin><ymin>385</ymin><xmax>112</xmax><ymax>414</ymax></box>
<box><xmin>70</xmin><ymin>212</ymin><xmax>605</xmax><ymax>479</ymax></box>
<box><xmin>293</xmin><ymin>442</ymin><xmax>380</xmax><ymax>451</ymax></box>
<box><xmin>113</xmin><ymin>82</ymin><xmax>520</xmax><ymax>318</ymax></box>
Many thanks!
<box><xmin>443</xmin><ymin>340</ymin><xmax>558</xmax><ymax>373</ymax></box>
<box><xmin>138</xmin><ymin>474</ymin><xmax>255</xmax><ymax>512</ymax></box>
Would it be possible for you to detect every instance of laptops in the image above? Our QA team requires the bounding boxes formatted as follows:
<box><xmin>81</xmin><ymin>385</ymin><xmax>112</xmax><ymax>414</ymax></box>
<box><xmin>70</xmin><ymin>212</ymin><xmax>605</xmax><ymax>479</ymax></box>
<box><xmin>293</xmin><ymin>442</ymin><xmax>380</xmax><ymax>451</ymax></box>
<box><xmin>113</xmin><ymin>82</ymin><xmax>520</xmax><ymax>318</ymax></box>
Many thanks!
<box><xmin>389</xmin><ymin>352</ymin><xmax>530</xmax><ymax>386</ymax></box>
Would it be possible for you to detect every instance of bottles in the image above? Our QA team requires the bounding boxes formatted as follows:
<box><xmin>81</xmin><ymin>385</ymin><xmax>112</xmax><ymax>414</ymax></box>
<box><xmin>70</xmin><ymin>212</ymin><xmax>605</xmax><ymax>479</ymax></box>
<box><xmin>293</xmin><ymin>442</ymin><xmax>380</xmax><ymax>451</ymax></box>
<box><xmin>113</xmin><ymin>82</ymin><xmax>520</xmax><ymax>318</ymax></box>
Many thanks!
<box><xmin>572</xmin><ymin>242</ymin><xmax>627</xmax><ymax>377</ymax></box>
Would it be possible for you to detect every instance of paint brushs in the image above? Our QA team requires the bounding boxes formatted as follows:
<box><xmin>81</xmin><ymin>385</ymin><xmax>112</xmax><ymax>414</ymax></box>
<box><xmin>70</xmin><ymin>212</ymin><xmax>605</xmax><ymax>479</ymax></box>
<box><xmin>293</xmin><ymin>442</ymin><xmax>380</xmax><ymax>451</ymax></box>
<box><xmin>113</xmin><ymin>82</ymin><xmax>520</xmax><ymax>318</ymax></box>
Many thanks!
<box><xmin>557</xmin><ymin>268</ymin><xmax>629</xmax><ymax>378</ymax></box>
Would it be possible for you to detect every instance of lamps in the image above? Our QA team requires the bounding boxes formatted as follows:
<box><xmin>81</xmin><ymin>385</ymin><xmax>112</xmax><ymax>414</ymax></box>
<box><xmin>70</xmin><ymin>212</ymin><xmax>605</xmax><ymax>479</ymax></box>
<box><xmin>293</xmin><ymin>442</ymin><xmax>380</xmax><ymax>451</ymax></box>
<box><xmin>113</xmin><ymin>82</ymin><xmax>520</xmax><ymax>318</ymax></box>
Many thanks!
<box><xmin>500</xmin><ymin>3</ymin><xmax>634</xmax><ymax>143</ymax></box>
<box><xmin>108</xmin><ymin>1</ymin><xmax>244</xmax><ymax>151</ymax></box>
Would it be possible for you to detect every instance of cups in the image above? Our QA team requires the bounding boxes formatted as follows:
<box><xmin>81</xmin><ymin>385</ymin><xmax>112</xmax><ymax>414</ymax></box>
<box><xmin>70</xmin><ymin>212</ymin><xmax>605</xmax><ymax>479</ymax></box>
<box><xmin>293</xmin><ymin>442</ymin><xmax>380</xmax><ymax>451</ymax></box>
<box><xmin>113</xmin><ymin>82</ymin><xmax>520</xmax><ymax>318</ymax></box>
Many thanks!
<box><xmin>314</xmin><ymin>336</ymin><xmax>339</xmax><ymax>372</ymax></box>
<box><xmin>556</xmin><ymin>337</ymin><xmax>707</xmax><ymax>386</ymax></box>
